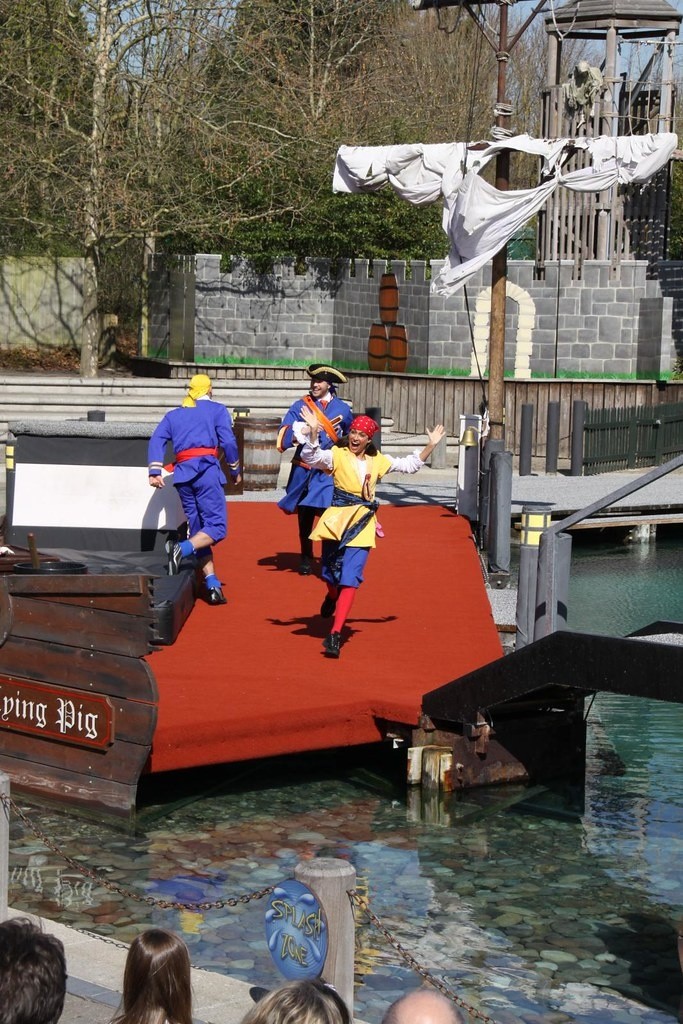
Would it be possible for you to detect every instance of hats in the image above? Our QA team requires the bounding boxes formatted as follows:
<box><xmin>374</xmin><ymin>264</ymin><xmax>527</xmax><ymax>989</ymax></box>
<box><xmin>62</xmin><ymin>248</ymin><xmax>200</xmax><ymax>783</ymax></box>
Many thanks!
<box><xmin>349</xmin><ymin>415</ymin><xmax>381</xmax><ymax>439</ymax></box>
<box><xmin>306</xmin><ymin>364</ymin><xmax>348</xmax><ymax>383</ymax></box>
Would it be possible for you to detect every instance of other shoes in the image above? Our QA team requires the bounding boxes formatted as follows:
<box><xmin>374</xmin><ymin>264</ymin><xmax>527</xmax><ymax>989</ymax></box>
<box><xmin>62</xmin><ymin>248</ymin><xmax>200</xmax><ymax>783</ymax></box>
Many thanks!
<box><xmin>300</xmin><ymin>562</ymin><xmax>311</xmax><ymax>574</ymax></box>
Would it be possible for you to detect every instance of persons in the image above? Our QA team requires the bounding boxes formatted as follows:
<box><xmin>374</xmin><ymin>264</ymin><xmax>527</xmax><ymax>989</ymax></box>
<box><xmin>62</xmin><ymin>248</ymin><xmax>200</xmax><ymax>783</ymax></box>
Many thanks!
<box><xmin>0</xmin><ymin>915</ymin><xmax>69</xmax><ymax>1024</ymax></box>
<box><xmin>148</xmin><ymin>373</ymin><xmax>242</xmax><ymax>605</ymax></box>
<box><xmin>276</xmin><ymin>364</ymin><xmax>354</xmax><ymax>574</ymax></box>
<box><xmin>380</xmin><ymin>991</ymin><xmax>466</xmax><ymax>1024</ymax></box>
<box><xmin>292</xmin><ymin>405</ymin><xmax>447</xmax><ymax>656</ymax></box>
<box><xmin>240</xmin><ymin>975</ymin><xmax>354</xmax><ymax>1024</ymax></box>
<box><xmin>108</xmin><ymin>929</ymin><xmax>207</xmax><ymax>1024</ymax></box>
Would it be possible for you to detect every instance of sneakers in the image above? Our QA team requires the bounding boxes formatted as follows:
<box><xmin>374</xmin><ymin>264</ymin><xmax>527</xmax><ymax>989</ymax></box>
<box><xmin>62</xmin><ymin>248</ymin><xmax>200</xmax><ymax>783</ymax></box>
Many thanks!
<box><xmin>207</xmin><ymin>586</ymin><xmax>227</xmax><ymax>606</ymax></box>
<box><xmin>322</xmin><ymin>630</ymin><xmax>342</xmax><ymax>656</ymax></box>
<box><xmin>320</xmin><ymin>586</ymin><xmax>341</xmax><ymax>618</ymax></box>
<box><xmin>165</xmin><ymin>540</ymin><xmax>182</xmax><ymax>577</ymax></box>
